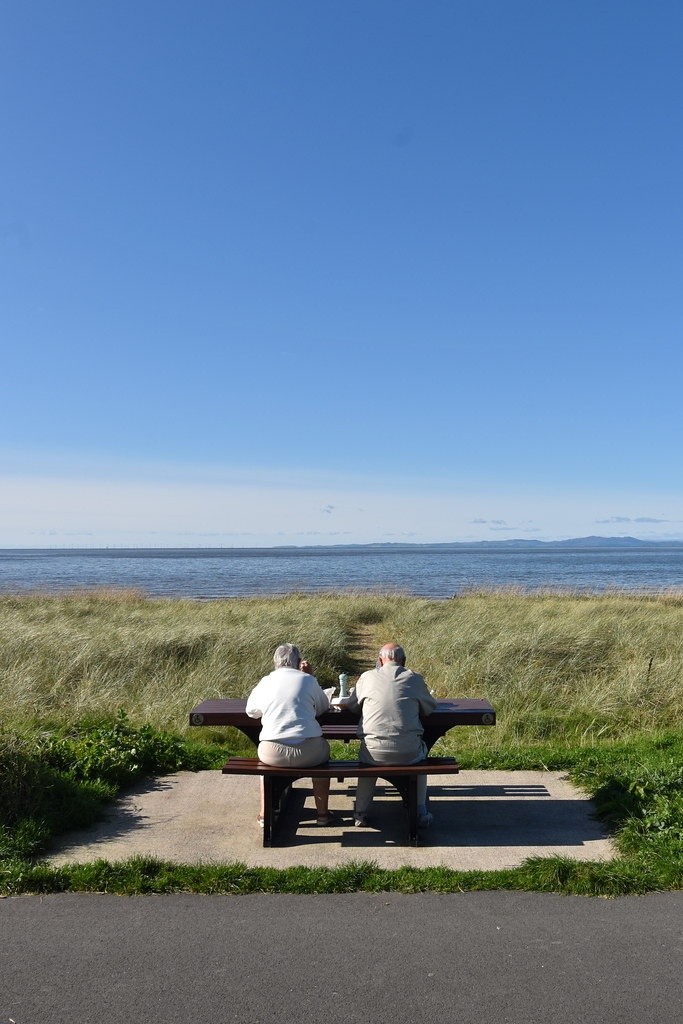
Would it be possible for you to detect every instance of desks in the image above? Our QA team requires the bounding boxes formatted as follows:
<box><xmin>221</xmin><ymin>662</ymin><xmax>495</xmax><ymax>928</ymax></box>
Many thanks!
<box><xmin>189</xmin><ymin>698</ymin><xmax>497</xmax><ymax>831</ymax></box>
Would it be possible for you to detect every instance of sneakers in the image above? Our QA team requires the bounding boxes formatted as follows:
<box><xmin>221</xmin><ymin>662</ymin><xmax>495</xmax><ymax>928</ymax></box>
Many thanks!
<box><xmin>257</xmin><ymin>814</ymin><xmax>265</xmax><ymax>827</ymax></box>
<box><xmin>418</xmin><ymin>811</ymin><xmax>434</xmax><ymax>828</ymax></box>
<box><xmin>317</xmin><ymin>811</ymin><xmax>334</xmax><ymax>825</ymax></box>
<box><xmin>354</xmin><ymin>818</ymin><xmax>367</xmax><ymax>827</ymax></box>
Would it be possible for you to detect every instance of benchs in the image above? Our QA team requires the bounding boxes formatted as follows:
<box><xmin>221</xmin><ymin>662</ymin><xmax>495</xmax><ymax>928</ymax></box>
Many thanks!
<box><xmin>319</xmin><ymin>723</ymin><xmax>363</xmax><ymax>740</ymax></box>
<box><xmin>220</xmin><ymin>758</ymin><xmax>461</xmax><ymax>848</ymax></box>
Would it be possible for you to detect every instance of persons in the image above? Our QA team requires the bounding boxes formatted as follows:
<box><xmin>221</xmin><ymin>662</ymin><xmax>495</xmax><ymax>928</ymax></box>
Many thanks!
<box><xmin>347</xmin><ymin>643</ymin><xmax>434</xmax><ymax>829</ymax></box>
<box><xmin>245</xmin><ymin>643</ymin><xmax>335</xmax><ymax>825</ymax></box>
